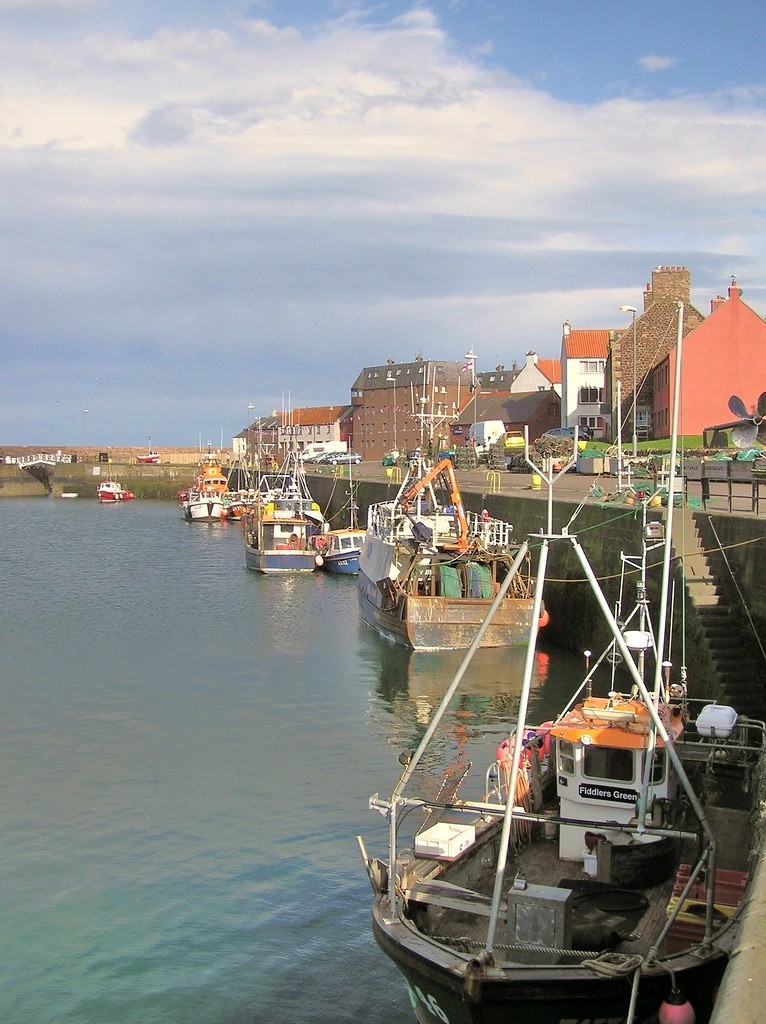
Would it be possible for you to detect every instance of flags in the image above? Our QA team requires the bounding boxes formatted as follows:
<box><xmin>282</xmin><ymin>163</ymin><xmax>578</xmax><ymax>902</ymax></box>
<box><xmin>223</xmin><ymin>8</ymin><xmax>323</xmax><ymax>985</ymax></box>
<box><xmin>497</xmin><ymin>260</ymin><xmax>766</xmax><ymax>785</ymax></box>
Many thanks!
<box><xmin>461</xmin><ymin>361</ymin><xmax>473</xmax><ymax>372</ymax></box>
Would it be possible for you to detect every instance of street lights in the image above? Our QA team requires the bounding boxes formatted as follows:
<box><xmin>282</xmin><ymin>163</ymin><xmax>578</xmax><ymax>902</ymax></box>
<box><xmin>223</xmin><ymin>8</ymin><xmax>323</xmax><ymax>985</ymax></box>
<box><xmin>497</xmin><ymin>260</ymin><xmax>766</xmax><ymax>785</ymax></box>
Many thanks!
<box><xmin>84</xmin><ymin>410</ymin><xmax>89</xmax><ymax>446</ymax></box>
<box><xmin>386</xmin><ymin>378</ymin><xmax>396</xmax><ymax>449</ymax></box>
<box><xmin>465</xmin><ymin>355</ymin><xmax>476</xmax><ymax>448</ymax></box>
<box><xmin>620</xmin><ymin>305</ymin><xmax>637</xmax><ymax>458</ymax></box>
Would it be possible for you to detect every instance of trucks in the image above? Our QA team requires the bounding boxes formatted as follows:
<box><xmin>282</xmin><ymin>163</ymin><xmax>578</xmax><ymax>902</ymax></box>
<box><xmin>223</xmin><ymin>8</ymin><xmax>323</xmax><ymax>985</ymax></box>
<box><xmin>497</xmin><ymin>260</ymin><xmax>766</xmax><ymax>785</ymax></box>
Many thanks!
<box><xmin>464</xmin><ymin>420</ymin><xmax>506</xmax><ymax>448</ymax></box>
<box><xmin>298</xmin><ymin>441</ymin><xmax>347</xmax><ymax>464</ymax></box>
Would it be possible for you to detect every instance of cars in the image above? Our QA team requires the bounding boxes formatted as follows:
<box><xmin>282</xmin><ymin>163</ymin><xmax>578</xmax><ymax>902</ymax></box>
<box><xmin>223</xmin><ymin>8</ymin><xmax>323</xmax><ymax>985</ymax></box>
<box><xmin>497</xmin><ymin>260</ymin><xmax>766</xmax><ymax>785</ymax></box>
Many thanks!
<box><xmin>570</xmin><ymin>426</ymin><xmax>594</xmax><ymax>439</ymax></box>
<box><xmin>326</xmin><ymin>452</ymin><xmax>362</xmax><ymax>464</ymax></box>
<box><xmin>495</xmin><ymin>431</ymin><xmax>525</xmax><ymax>449</ymax></box>
<box><xmin>307</xmin><ymin>452</ymin><xmax>337</xmax><ymax>464</ymax></box>
<box><xmin>545</xmin><ymin>428</ymin><xmax>589</xmax><ymax>442</ymax></box>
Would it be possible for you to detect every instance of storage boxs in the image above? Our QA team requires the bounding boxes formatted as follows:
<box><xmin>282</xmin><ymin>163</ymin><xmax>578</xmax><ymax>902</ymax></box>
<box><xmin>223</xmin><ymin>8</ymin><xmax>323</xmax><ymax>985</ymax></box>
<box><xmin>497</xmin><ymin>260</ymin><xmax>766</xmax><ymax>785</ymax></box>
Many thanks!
<box><xmin>415</xmin><ymin>822</ymin><xmax>476</xmax><ymax>858</ymax></box>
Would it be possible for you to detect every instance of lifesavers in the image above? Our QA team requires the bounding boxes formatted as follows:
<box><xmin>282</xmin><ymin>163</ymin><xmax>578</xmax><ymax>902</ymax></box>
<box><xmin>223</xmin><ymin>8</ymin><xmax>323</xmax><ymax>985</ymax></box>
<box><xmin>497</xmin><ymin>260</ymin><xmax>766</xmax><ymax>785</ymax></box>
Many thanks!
<box><xmin>480</xmin><ymin>509</ymin><xmax>488</xmax><ymax>528</ymax></box>
<box><xmin>613</xmin><ymin>701</ymin><xmax>671</xmax><ymax>735</ymax></box>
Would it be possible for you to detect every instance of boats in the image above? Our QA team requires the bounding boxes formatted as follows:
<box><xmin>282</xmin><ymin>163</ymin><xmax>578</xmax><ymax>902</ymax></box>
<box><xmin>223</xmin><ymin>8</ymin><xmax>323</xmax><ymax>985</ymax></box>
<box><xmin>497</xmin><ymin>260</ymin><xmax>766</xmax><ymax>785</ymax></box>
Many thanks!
<box><xmin>221</xmin><ymin>452</ymin><xmax>257</xmax><ymax>521</ymax></box>
<box><xmin>245</xmin><ymin>452</ymin><xmax>323</xmax><ymax>574</ymax></box>
<box><xmin>96</xmin><ymin>481</ymin><xmax>134</xmax><ymax>502</ymax></box>
<box><xmin>177</xmin><ymin>488</ymin><xmax>190</xmax><ymax>509</ymax></box>
<box><xmin>179</xmin><ymin>458</ymin><xmax>229</xmax><ymax>522</ymax></box>
<box><xmin>354</xmin><ymin>422</ymin><xmax>766</xmax><ymax>1024</ymax></box>
<box><xmin>137</xmin><ymin>451</ymin><xmax>160</xmax><ymax>463</ymax></box>
<box><xmin>358</xmin><ymin>366</ymin><xmax>544</xmax><ymax>653</ymax></box>
<box><xmin>307</xmin><ymin>480</ymin><xmax>367</xmax><ymax>574</ymax></box>
